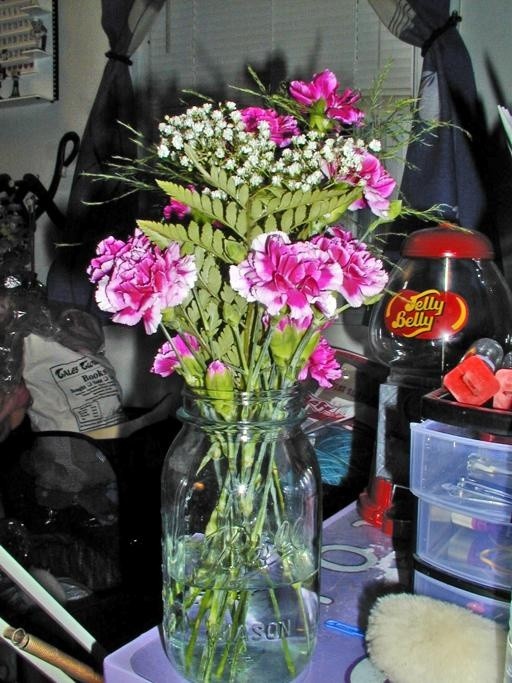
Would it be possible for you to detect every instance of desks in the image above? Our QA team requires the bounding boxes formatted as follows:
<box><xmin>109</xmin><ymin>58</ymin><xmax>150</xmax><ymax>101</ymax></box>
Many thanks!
<box><xmin>100</xmin><ymin>500</ymin><xmax>415</xmax><ymax>680</ymax></box>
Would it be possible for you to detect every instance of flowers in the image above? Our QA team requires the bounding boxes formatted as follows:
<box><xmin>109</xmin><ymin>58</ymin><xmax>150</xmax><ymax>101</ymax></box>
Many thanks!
<box><xmin>56</xmin><ymin>64</ymin><xmax>476</xmax><ymax>682</ymax></box>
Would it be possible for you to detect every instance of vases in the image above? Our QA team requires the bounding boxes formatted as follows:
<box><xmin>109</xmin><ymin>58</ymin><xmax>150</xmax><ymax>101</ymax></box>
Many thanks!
<box><xmin>158</xmin><ymin>387</ymin><xmax>325</xmax><ymax>683</ymax></box>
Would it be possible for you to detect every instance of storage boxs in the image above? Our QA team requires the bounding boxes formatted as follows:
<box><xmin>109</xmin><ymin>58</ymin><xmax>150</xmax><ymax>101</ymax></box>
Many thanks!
<box><xmin>409</xmin><ymin>415</ymin><xmax>512</xmax><ymax>623</ymax></box>
